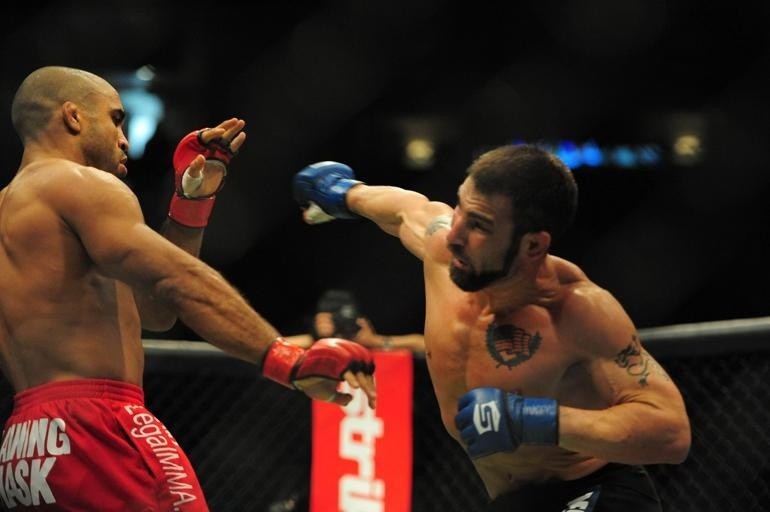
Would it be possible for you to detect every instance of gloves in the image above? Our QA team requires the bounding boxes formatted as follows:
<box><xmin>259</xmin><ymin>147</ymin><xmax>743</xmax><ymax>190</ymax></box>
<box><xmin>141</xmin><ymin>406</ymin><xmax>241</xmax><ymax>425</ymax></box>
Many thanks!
<box><xmin>293</xmin><ymin>161</ymin><xmax>365</xmax><ymax>225</ymax></box>
<box><xmin>455</xmin><ymin>387</ymin><xmax>558</xmax><ymax>460</ymax></box>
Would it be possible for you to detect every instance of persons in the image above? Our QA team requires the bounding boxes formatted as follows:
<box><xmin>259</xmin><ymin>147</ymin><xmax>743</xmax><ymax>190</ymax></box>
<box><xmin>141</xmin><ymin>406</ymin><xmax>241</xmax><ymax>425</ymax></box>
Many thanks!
<box><xmin>293</xmin><ymin>144</ymin><xmax>692</xmax><ymax>512</ymax></box>
<box><xmin>0</xmin><ymin>66</ymin><xmax>378</xmax><ymax>512</ymax></box>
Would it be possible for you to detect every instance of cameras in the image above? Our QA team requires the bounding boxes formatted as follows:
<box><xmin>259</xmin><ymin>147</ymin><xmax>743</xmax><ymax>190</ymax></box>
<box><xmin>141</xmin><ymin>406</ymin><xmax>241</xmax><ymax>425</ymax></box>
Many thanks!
<box><xmin>333</xmin><ymin>303</ymin><xmax>360</xmax><ymax>340</ymax></box>
